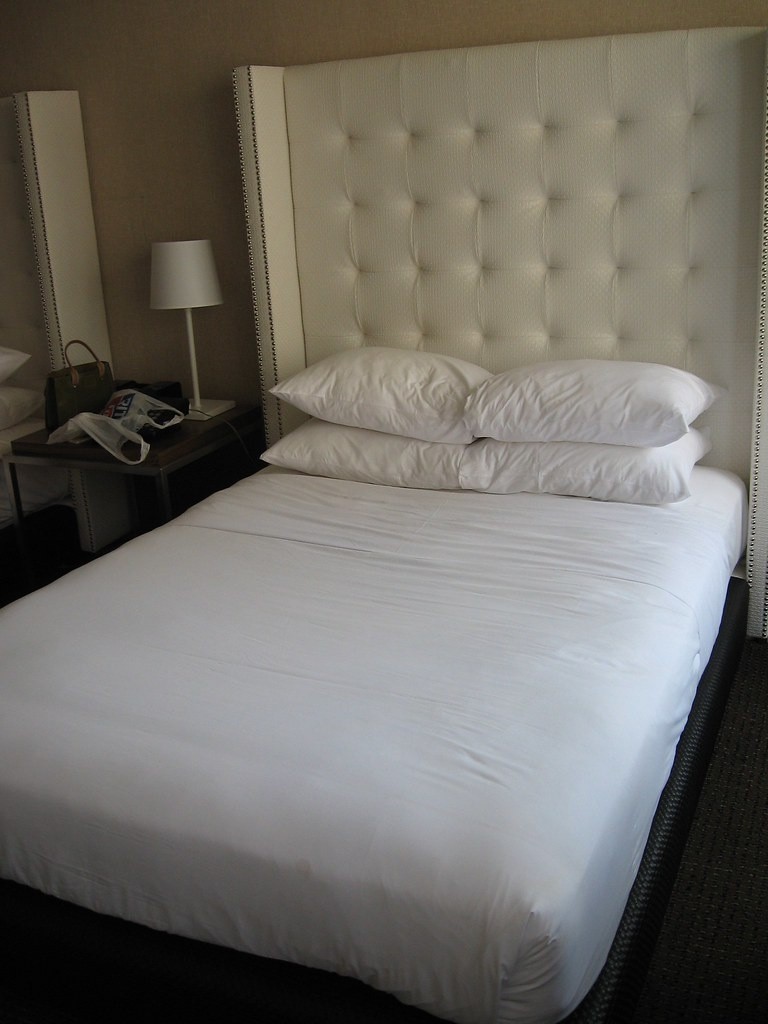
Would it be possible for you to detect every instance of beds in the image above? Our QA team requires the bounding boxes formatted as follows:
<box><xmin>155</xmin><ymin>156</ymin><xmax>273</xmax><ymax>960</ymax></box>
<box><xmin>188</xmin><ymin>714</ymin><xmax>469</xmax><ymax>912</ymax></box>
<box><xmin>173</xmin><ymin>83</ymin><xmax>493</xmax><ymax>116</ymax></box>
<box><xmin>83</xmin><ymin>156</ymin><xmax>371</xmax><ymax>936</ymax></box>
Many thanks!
<box><xmin>0</xmin><ymin>462</ymin><xmax>754</xmax><ymax>1024</ymax></box>
<box><xmin>0</xmin><ymin>90</ymin><xmax>117</xmax><ymax>529</ymax></box>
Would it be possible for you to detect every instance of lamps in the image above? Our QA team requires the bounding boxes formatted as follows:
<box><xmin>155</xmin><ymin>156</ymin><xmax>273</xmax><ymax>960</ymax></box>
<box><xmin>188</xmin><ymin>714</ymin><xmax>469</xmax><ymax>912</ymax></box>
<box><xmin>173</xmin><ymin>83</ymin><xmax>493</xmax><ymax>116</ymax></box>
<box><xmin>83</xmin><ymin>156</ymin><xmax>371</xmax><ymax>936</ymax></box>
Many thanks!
<box><xmin>149</xmin><ymin>240</ymin><xmax>237</xmax><ymax>420</ymax></box>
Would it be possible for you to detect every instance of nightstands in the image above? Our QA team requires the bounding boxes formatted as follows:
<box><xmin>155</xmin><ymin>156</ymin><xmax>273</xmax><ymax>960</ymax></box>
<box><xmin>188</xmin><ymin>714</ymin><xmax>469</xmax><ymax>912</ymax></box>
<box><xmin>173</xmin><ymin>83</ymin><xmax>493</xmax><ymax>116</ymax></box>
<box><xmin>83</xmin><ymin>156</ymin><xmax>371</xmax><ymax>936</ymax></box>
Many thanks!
<box><xmin>3</xmin><ymin>394</ymin><xmax>263</xmax><ymax>576</ymax></box>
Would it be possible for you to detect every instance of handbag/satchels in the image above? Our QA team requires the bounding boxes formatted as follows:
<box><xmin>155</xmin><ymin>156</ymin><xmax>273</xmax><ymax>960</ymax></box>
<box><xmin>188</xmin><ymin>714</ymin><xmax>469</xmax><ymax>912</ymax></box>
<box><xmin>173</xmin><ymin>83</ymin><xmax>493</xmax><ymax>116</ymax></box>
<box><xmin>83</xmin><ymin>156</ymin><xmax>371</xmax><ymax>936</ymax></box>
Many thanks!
<box><xmin>48</xmin><ymin>389</ymin><xmax>185</xmax><ymax>465</ymax></box>
<box><xmin>43</xmin><ymin>340</ymin><xmax>115</xmax><ymax>436</ymax></box>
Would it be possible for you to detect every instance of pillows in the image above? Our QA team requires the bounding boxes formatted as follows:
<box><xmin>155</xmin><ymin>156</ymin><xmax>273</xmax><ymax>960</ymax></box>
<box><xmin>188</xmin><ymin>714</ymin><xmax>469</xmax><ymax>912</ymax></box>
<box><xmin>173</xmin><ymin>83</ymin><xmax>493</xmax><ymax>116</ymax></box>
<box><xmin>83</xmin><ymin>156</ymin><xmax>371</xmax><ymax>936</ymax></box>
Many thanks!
<box><xmin>0</xmin><ymin>348</ymin><xmax>32</xmax><ymax>384</ymax></box>
<box><xmin>269</xmin><ymin>347</ymin><xmax>494</xmax><ymax>444</ymax></box>
<box><xmin>461</xmin><ymin>360</ymin><xmax>728</xmax><ymax>449</ymax></box>
<box><xmin>258</xmin><ymin>417</ymin><xmax>472</xmax><ymax>491</ymax></box>
<box><xmin>459</xmin><ymin>424</ymin><xmax>713</xmax><ymax>505</ymax></box>
<box><xmin>0</xmin><ymin>386</ymin><xmax>46</xmax><ymax>432</ymax></box>
